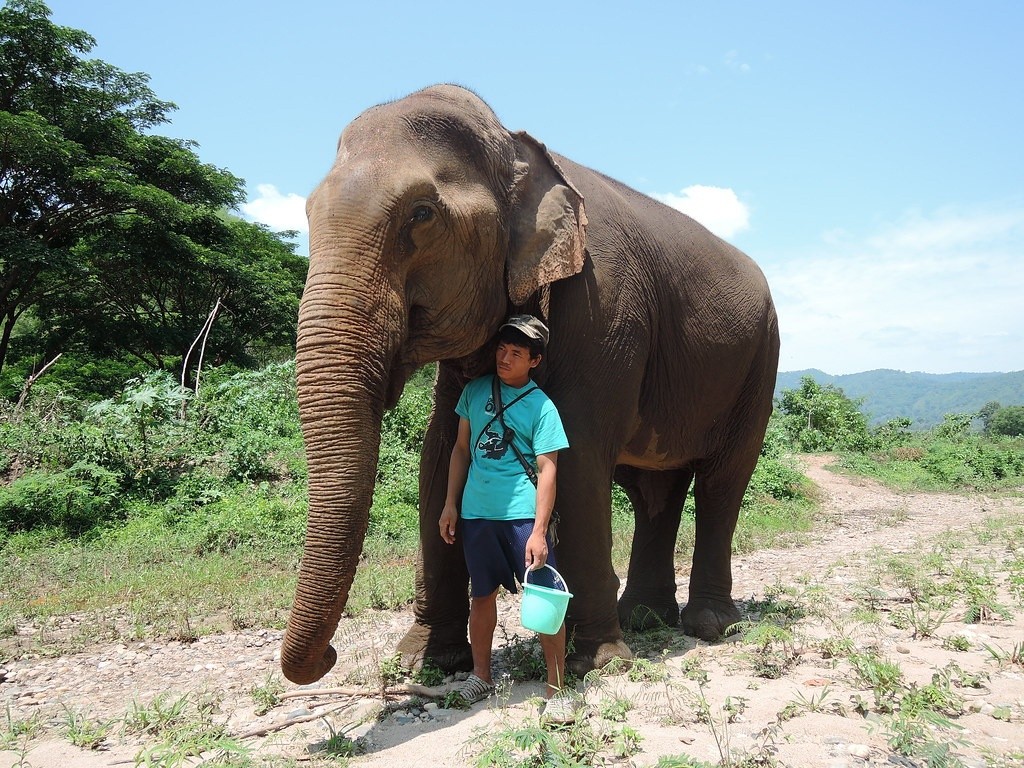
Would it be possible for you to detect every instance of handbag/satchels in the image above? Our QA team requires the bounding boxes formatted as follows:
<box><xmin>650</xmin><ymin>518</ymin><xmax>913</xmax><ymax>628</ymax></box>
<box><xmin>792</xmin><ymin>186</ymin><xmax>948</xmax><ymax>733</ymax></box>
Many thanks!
<box><xmin>548</xmin><ymin>508</ymin><xmax>560</xmax><ymax>549</ymax></box>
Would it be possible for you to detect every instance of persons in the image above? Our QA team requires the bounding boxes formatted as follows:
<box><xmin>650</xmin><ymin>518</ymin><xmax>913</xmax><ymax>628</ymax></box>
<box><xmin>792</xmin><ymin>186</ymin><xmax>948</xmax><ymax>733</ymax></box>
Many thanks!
<box><xmin>439</xmin><ymin>312</ymin><xmax>579</xmax><ymax>730</ymax></box>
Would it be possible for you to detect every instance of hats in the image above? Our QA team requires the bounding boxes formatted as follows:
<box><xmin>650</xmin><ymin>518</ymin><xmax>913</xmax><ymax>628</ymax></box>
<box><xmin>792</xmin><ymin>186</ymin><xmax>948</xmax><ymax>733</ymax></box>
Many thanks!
<box><xmin>499</xmin><ymin>314</ymin><xmax>549</xmax><ymax>348</ymax></box>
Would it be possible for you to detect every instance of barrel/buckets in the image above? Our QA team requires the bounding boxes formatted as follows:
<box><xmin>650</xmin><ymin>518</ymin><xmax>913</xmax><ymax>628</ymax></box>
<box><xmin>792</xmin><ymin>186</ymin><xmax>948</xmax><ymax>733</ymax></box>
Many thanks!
<box><xmin>521</xmin><ymin>563</ymin><xmax>573</xmax><ymax>635</ymax></box>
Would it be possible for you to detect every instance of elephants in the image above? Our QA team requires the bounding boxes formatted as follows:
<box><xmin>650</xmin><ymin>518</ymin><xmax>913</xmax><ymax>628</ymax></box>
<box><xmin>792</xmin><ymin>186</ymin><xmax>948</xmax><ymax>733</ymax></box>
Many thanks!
<box><xmin>280</xmin><ymin>83</ymin><xmax>781</xmax><ymax>685</ymax></box>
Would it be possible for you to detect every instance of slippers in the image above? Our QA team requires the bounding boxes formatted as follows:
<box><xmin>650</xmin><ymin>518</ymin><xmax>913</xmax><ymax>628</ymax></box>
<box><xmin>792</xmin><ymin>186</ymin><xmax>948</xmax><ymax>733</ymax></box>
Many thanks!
<box><xmin>456</xmin><ymin>674</ymin><xmax>501</xmax><ymax>705</ymax></box>
<box><xmin>542</xmin><ymin>699</ymin><xmax>575</xmax><ymax>724</ymax></box>
